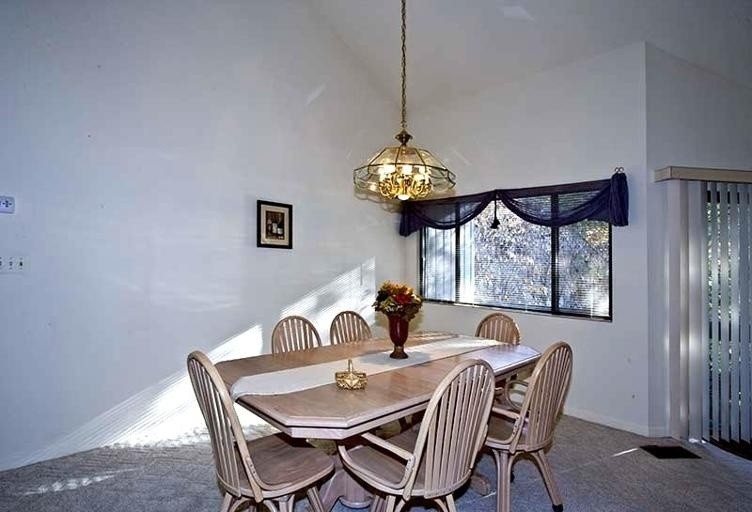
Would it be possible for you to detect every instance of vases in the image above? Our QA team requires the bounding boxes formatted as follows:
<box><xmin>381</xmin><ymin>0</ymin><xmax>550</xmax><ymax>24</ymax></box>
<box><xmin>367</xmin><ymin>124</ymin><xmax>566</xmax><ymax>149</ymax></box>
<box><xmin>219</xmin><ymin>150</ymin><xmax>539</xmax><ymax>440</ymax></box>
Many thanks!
<box><xmin>381</xmin><ymin>310</ymin><xmax>412</xmax><ymax>361</ymax></box>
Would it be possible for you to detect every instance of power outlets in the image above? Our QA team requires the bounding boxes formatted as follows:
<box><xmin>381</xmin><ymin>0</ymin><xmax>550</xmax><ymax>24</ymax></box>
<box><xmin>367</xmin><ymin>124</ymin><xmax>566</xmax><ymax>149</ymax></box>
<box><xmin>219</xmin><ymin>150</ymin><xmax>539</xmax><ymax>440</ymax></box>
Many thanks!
<box><xmin>0</xmin><ymin>195</ymin><xmax>16</xmax><ymax>214</ymax></box>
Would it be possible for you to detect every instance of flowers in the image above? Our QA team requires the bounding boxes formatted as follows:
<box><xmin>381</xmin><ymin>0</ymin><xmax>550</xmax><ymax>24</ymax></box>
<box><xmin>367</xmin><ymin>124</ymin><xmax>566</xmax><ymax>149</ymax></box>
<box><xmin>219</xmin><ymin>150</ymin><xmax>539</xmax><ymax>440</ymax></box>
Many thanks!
<box><xmin>372</xmin><ymin>280</ymin><xmax>421</xmax><ymax>323</ymax></box>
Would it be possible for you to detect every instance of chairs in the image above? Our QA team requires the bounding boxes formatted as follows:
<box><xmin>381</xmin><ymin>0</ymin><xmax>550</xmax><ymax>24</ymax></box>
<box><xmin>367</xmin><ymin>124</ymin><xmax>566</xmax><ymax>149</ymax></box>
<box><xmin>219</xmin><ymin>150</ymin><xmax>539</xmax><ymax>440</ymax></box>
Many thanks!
<box><xmin>335</xmin><ymin>359</ymin><xmax>494</xmax><ymax>511</ymax></box>
<box><xmin>471</xmin><ymin>342</ymin><xmax>573</xmax><ymax>511</ymax></box>
<box><xmin>474</xmin><ymin>313</ymin><xmax>520</xmax><ymax>348</ymax></box>
<box><xmin>271</xmin><ymin>316</ymin><xmax>322</xmax><ymax>351</ymax></box>
<box><xmin>186</xmin><ymin>350</ymin><xmax>334</xmax><ymax>511</ymax></box>
<box><xmin>330</xmin><ymin>312</ymin><xmax>369</xmax><ymax>342</ymax></box>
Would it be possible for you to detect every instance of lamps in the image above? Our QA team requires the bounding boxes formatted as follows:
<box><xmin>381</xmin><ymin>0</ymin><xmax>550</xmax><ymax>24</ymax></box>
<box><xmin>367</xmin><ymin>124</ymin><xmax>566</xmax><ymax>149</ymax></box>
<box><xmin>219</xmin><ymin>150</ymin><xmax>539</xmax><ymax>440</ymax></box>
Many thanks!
<box><xmin>349</xmin><ymin>0</ymin><xmax>456</xmax><ymax>200</ymax></box>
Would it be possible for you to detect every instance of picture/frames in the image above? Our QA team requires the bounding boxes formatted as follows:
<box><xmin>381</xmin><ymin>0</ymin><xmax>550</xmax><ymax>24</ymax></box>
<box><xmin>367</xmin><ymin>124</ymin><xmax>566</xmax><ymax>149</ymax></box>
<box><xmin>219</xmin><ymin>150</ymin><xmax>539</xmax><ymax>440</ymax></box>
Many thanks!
<box><xmin>258</xmin><ymin>200</ymin><xmax>294</xmax><ymax>249</ymax></box>
